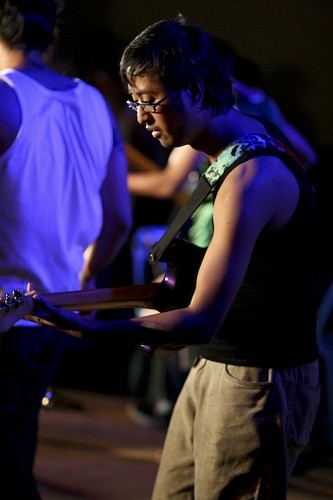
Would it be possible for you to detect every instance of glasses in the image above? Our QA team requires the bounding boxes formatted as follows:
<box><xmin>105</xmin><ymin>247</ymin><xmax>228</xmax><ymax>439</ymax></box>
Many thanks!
<box><xmin>126</xmin><ymin>93</ymin><xmax>180</xmax><ymax>113</ymax></box>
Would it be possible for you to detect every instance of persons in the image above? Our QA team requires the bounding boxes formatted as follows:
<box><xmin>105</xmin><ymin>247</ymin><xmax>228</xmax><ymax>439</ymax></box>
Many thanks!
<box><xmin>23</xmin><ymin>16</ymin><xmax>333</xmax><ymax>500</ymax></box>
<box><xmin>0</xmin><ymin>0</ymin><xmax>134</xmax><ymax>500</ymax></box>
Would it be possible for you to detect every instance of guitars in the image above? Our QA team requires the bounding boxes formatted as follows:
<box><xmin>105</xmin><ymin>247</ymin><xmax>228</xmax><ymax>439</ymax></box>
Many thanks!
<box><xmin>0</xmin><ymin>237</ymin><xmax>206</xmax><ymax>332</ymax></box>
